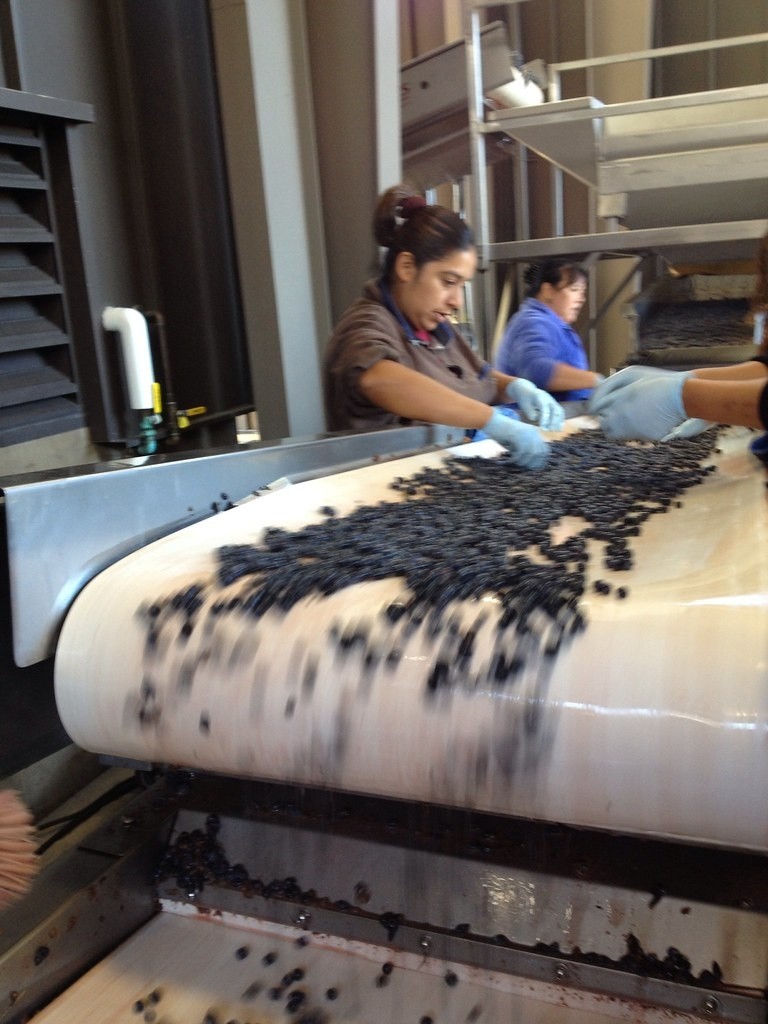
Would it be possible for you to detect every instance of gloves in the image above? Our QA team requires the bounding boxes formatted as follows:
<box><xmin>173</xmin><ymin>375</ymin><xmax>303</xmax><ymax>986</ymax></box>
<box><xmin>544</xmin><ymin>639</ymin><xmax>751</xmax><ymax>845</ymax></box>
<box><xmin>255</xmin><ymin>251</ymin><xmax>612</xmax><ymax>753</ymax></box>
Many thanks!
<box><xmin>505</xmin><ymin>379</ymin><xmax>565</xmax><ymax>432</ymax></box>
<box><xmin>588</xmin><ymin>365</ymin><xmax>695</xmax><ymax>442</ymax></box>
<box><xmin>480</xmin><ymin>412</ymin><xmax>551</xmax><ymax>470</ymax></box>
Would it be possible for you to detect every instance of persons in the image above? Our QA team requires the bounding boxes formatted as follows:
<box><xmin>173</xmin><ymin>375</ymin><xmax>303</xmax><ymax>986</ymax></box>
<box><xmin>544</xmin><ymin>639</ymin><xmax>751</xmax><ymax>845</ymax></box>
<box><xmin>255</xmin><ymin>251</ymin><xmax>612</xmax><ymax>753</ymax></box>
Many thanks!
<box><xmin>585</xmin><ymin>310</ymin><xmax>768</xmax><ymax>498</ymax></box>
<box><xmin>322</xmin><ymin>181</ymin><xmax>567</xmax><ymax>473</ymax></box>
<box><xmin>494</xmin><ymin>259</ymin><xmax>610</xmax><ymax>409</ymax></box>
